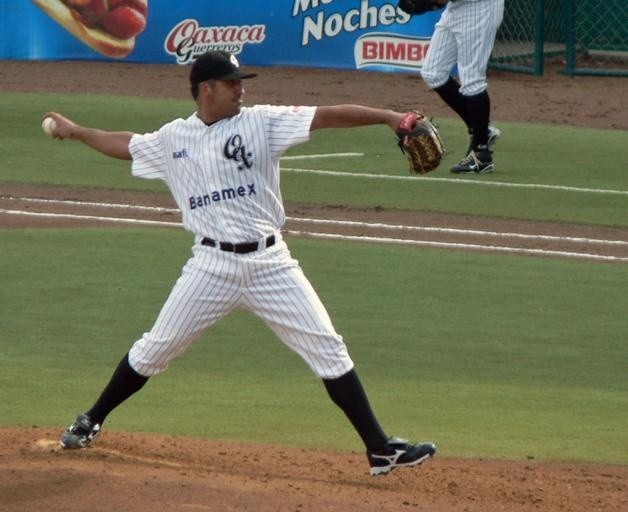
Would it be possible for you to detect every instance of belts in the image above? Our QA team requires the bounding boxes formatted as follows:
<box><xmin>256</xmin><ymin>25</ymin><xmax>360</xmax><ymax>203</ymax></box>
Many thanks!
<box><xmin>199</xmin><ymin>235</ymin><xmax>275</xmax><ymax>254</ymax></box>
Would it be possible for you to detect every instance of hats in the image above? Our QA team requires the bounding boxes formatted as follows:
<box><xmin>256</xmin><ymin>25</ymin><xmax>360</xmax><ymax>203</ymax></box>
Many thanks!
<box><xmin>189</xmin><ymin>50</ymin><xmax>258</xmax><ymax>83</ymax></box>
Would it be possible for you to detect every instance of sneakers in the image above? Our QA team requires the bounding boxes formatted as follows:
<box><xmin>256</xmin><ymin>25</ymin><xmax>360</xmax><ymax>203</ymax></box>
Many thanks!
<box><xmin>449</xmin><ymin>150</ymin><xmax>495</xmax><ymax>173</ymax></box>
<box><xmin>59</xmin><ymin>412</ymin><xmax>99</xmax><ymax>448</ymax></box>
<box><xmin>366</xmin><ymin>435</ymin><xmax>436</xmax><ymax>477</ymax></box>
<box><xmin>466</xmin><ymin>126</ymin><xmax>501</xmax><ymax>156</ymax></box>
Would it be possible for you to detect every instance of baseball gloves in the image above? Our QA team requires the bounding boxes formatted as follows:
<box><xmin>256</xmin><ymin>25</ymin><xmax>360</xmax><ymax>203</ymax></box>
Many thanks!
<box><xmin>394</xmin><ymin>110</ymin><xmax>443</xmax><ymax>175</ymax></box>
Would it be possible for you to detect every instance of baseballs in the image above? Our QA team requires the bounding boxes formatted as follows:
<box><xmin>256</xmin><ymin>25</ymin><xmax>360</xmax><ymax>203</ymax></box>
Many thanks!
<box><xmin>41</xmin><ymin>113</ymin><xmax>59</xmax><ymax>135</ymax></box>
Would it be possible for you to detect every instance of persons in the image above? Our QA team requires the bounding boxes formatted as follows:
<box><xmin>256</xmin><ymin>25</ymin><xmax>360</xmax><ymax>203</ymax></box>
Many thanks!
<box><xmin>42</xmin><ymin>48</ymin><xmax>439</xmax><ymax>477</ymax></box>
<box><xmin>398</xmin><ymin>0</ymin><xmax>503</xmax><ymax>177</ymax></box>
